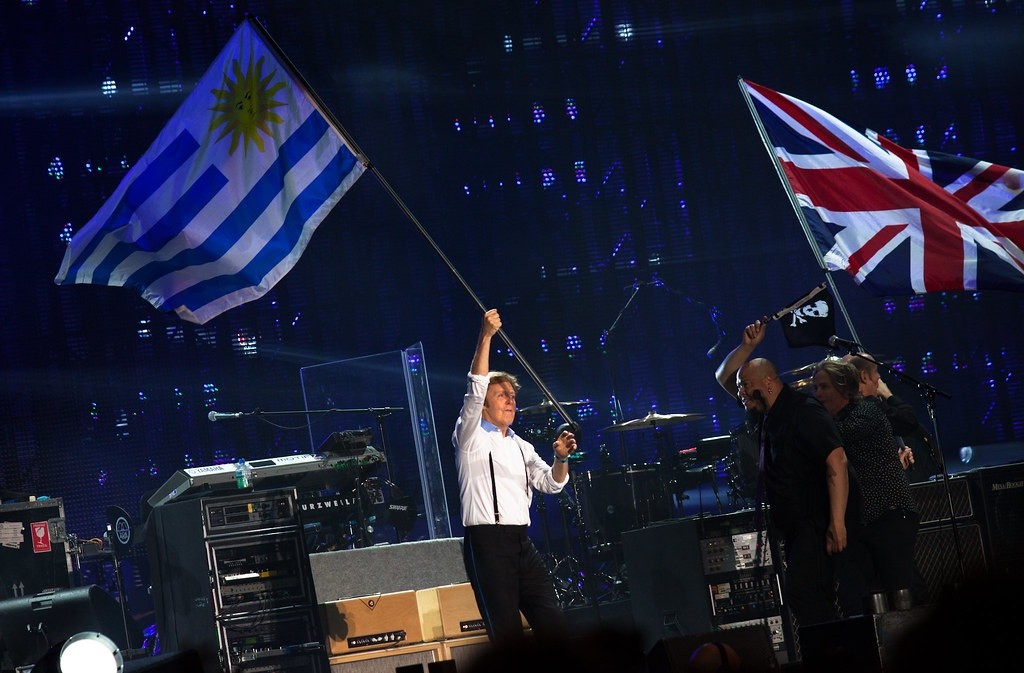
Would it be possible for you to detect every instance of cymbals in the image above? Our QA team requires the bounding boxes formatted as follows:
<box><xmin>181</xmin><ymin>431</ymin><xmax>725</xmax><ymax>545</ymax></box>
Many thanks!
<box><xmin>519</xmin><ymin>400</ymin><xmax>588</xmax><ymax>413</ymax></box>
<box><xmin>596</xmin><ymin>412</ymin><xmax>707</xmax><ymax>432</ymax></box>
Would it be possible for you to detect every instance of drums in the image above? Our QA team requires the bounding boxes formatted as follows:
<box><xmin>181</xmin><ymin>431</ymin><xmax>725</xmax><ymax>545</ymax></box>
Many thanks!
<box><xmin>576</xmin><ymin>465</ymin><xmax>677</xmax><ymax>557</ymax></box>
<box><xmin>781</xmin><ymin>360</ymin><xmax>822</xmax><ymax>396</ymax></box>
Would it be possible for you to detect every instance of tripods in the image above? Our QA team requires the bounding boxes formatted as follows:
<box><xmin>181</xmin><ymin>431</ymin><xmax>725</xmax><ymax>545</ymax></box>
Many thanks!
<box><xmin>536</xmin><ymin>493</ymin><xmax>628</xmax><ymax>608</ymax></box>
<box><xmin>721</xmin><ymin>457</ymin><xmax>751</xmax><ymax>511</ymax></box>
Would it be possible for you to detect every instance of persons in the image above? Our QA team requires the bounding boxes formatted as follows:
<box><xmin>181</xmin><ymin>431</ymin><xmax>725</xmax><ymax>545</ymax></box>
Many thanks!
<box><xmin>744</xmin><ymin>80</ymin><xmax>1024</xmax><ymax>296</ymax></box>
<box><xmin>714</xmin><ymin>315</ymin><xmax>849</xmax><ymax>673</ymax></box>
<box><xmin>814</xmin><ymin>352</ymin><xmax>929</xmax><ymax>607</ymax></box>
<box><xmin>451</xmin><ymin>308</ymin><xmax>577</xmax><ymax>643</ymax></box>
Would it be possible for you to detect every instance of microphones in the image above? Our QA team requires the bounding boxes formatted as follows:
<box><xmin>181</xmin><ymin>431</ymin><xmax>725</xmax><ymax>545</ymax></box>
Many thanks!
<box><xmin>829</xmin><ymin>336</ymin><xmax>861</xmax><ymax>348</ymax></box>
<box><xmin>208</xmin><ymin>411</ymin><xmax>243</xmax><ymax>422</ymax></box>
<box><xmin>623</xmin><ymin>280</ymin><xmax>662</xmax><ymax>290</ymax></box>
<box><xmin>752</xmin><ymin>389</ymin><xmax>768</xmax><ymax>409</ymax></box>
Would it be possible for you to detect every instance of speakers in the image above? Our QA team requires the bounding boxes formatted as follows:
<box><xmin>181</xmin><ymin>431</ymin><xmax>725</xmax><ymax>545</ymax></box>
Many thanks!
<box><xmin>415</xmin><ymin>582</ymin><xmax>531</xmax><ymax>642</ymax></box>
<box><xmin>0</xmin><ymin>584</ymin><xmax>145</xmax><ymax>673</ymax></box>
<box><xmin>320</xmin><ymin>589</ymin><xmax>423</xmax><ymax>655</ymax></box>
<box><xmin>622</xmin><ymin>461</ymin><xmax>1024</xmax><ymax>673</ymax></box>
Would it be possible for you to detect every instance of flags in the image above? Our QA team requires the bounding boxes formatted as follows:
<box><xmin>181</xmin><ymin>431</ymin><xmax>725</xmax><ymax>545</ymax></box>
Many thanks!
<box><xmin>53</xmin><ymin>19</ymin><xmax>366</xmax><ymax>324</ymax></box>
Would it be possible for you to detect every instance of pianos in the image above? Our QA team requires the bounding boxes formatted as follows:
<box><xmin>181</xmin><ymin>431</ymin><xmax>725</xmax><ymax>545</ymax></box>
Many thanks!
<box><xmin>147</xmin><ymin>445</ymin><xmax>386</xmax><ymax>508</ymax></box>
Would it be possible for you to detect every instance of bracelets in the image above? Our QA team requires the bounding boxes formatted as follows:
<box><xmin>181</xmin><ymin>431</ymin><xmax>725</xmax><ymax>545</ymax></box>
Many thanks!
<box><xmin>555</xmin><ymin>455</ymin><xmax>567</xmax><ymax>463</ymax></box>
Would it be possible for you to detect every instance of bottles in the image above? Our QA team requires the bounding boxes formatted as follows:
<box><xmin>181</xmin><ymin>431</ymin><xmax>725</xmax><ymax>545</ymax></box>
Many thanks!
<box><xmin>234</xmin><ymin>458</ymin><xmax>254</xmax><ymax>490</ymax></box>
